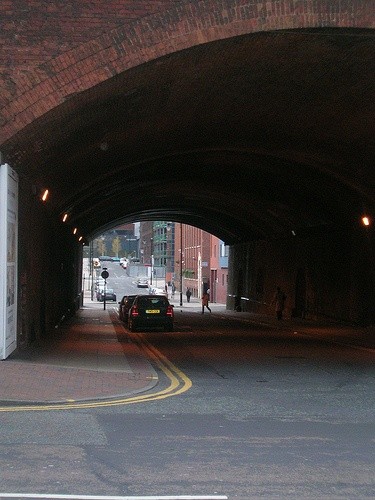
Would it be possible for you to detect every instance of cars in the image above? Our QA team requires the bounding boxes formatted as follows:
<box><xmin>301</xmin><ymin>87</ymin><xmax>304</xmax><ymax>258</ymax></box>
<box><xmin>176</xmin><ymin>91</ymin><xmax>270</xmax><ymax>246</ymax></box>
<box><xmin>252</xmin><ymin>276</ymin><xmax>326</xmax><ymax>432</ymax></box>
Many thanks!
<box><xmin>128</xmin><ymin>294</ymin><xmax>174</xmax><ymax>332</ymax></box>
<box><xmin>110</xmin><ymin>256</ymin><xmax>140</xmax><ymax>269</ymax></box>
<box><xmin>99</xmin><ymin>255</ymin><xmax>111</xmax><ymax>261</ymax></box>
<box><xmin>93</xmin><ymin>258</ymin><xmax>116</xmax><ymax>302</ymax></box>
<box><xmin>117</xmin><ymin>295</ymin><xmax>138</xmax><ymax>324</ymax></box>
<box><xmin>149</xmin><ymin>287</ymin><xmax>168</xmax><ymax>299</ymax></box>
<box><xmin>136</xmin><ymin>279</ymin><xmax>148</xmax><ymax>288</ymax></box>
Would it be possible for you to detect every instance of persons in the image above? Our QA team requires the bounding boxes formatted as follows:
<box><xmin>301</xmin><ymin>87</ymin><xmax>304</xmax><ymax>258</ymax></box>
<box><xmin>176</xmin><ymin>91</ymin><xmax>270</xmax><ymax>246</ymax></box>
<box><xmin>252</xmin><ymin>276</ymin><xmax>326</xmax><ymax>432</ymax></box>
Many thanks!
<box><xmin>201</xmin><ymin>290</ymin><xmax>211</xmax><ymax>314</ymax></box>
<box><xmin>186</xmin><ymin>289</ymin><xmax>191</xmax><ymax>302</ymax></box>
<box><xmin>272</xmin><ymin>288</ymin><xmax>286</xmax><ymax>320</ymax></box>
